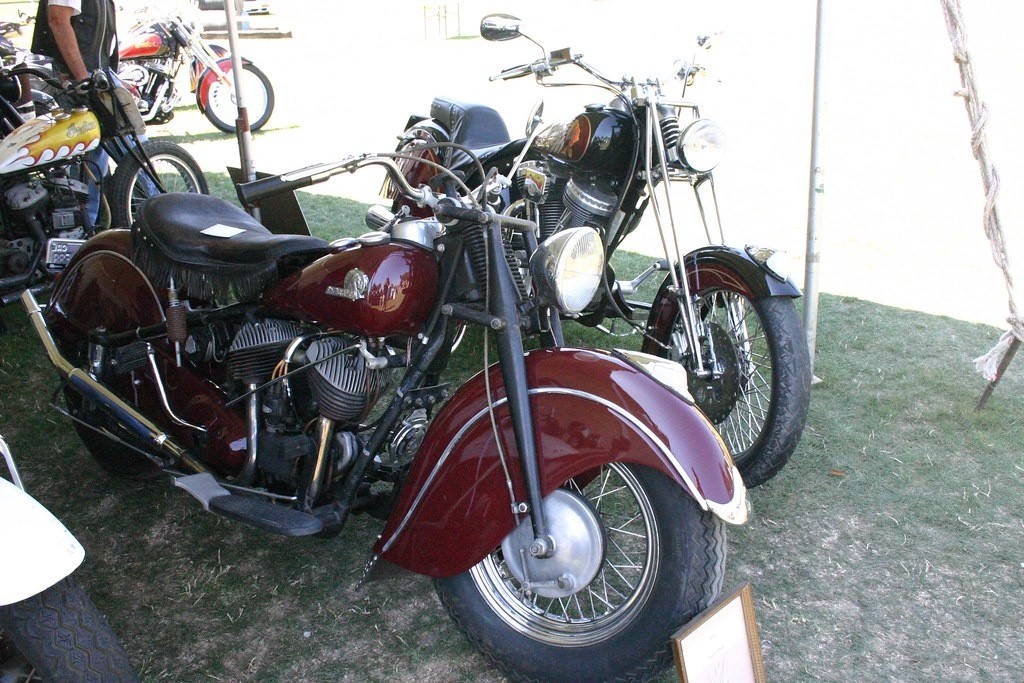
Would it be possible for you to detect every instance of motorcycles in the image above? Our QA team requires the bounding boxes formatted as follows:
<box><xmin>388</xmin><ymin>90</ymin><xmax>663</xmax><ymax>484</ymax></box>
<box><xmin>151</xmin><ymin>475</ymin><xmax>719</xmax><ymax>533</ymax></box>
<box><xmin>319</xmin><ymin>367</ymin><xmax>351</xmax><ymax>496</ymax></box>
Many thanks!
<box><xmin>0</xmin><ymin>8</ymin><xmax>274</xmax><ymax>134</ymax></box>
<box><xmin>367</xmin><ymin>13</ymin><xmax>812</xmax><ymax>492</ymax></box>
<box><xmin>0</xmin><ymin>58</ymin><xmax>209</xmax><ymax>312</ymax></box>
<box><xmin>17</xmin><ymin>121</ymin><xmax>752</xmax><ymax>683</ymax></box>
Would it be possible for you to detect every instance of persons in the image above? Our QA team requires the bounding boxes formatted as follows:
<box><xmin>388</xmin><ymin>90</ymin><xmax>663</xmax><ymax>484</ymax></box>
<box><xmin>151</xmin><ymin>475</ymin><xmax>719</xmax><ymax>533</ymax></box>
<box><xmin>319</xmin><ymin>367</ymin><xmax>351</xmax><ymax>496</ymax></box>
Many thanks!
<box><xmin>30</xmin><ymin>0</ymin><xmax>167</xmax><ymax>239</ymax></box>
